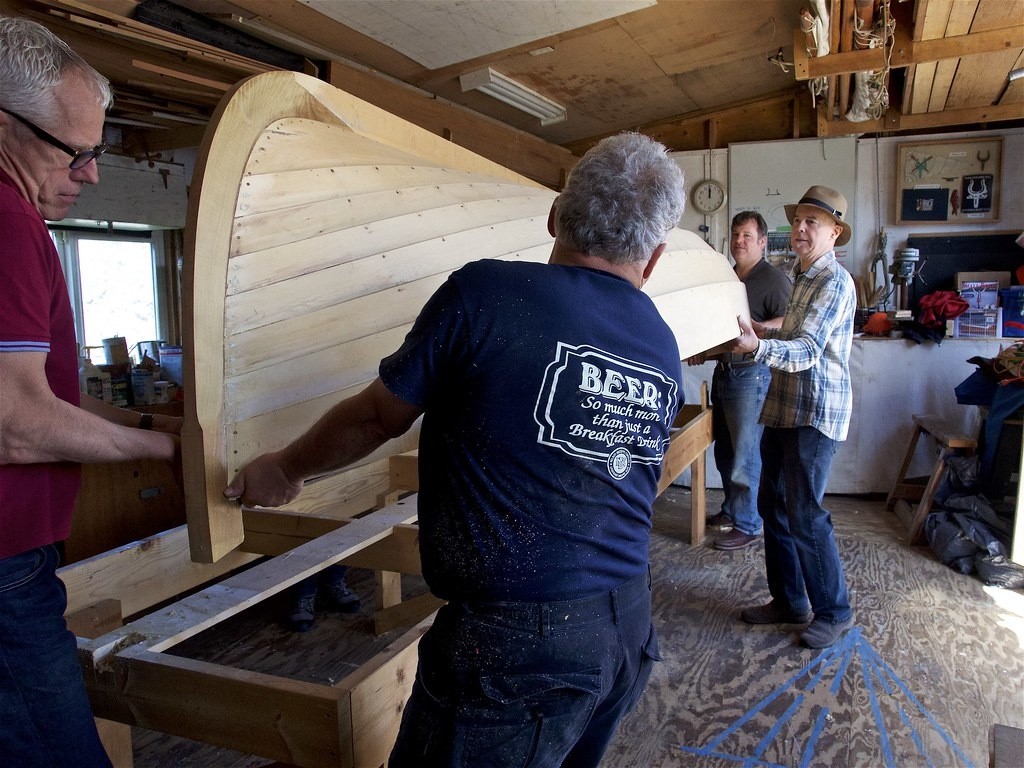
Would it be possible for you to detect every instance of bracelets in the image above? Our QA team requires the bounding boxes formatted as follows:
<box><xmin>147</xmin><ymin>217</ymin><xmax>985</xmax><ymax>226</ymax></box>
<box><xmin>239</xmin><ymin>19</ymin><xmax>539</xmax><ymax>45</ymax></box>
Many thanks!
<box><xmin>138</xmin><ymin>411</ymin><xmax>153</xmax><ymax>430</ymax></box>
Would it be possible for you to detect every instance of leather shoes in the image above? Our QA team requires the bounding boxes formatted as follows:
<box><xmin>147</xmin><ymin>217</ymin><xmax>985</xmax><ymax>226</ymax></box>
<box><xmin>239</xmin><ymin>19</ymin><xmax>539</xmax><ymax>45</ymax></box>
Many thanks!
<box><xmin>704</xmin><ymin>512</ymin><xmax>733</xmax><ymax>528</ymax></box>
<box><xmin>713</xmin><ymin>528</ymin><xmax>761</xmax><ymax>551</ymax></box>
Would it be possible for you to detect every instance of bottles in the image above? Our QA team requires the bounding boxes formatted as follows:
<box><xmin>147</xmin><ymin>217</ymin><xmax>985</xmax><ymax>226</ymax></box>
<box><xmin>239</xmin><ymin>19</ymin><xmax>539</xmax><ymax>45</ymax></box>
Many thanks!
<box><xmin>79</xmin><ymin>359</ymin><xmax>105</xmax><ymax>401</ymax></box>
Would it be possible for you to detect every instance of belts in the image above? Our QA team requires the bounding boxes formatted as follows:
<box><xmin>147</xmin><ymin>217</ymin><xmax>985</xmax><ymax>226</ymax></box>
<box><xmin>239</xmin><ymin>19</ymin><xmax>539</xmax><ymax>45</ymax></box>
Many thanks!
<box><xmin>718</xmin><ymin>360</ymin><xmax>756</xmax><ymax>372</ymax></box>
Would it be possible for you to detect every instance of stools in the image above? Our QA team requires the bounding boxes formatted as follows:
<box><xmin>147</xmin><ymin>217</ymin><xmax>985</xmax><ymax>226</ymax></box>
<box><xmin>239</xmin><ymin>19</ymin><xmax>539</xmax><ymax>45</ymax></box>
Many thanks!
<box><xmin>883</xmin><ymin>411</ymin><xmax>981</xmax><ymax>547</ymax></box>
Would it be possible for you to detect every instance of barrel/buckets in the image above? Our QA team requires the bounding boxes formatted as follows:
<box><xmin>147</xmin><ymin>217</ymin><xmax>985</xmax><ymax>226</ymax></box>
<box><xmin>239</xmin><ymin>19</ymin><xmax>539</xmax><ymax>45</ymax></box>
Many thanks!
<box><xmin>102</xmin><ymin>337</ymin><xmax>129</xmax><ymax>364</ymax></box>
<box><xmin>131</xmin><ymin>369</ymin><xmax>156</xmax><ymax>406</ymax></box>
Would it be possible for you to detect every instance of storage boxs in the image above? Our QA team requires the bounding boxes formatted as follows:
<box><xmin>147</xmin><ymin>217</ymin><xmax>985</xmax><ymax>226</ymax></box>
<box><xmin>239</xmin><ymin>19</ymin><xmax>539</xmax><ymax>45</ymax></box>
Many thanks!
<box><xmin>998</xmin><ymin>284</ymin><xmax>1024</xmax><ymax>338</ymax></box>
<box><xmin>130</xmin><ymin>368</ymin><xmax>156</xmax><ymax>407</ymax></box>
<box><xmin>96</xmin><ymin>363</ymin><xmax>134</xmax><ymax>407</ymax></box>
<box><xmin>160</xmin><ymin>345</ymin><xmax>185</xmax><ymax>400</ymax></box>
<box><xmin>154</xmin><ymin>381</ymin><xmax>169</xmax><ymax>404</ymax></box>
<box><xmin>958</xmin><ymin>279</ymin><xmax>1002</xmax><ymax>335</ymax></box>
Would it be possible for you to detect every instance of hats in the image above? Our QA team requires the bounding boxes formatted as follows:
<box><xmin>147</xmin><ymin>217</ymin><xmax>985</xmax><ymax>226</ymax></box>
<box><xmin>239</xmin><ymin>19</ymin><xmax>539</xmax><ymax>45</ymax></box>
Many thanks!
<box><xmin>784</xmin><ymin>186</ymin><xmax>851</xmax><ymax>246</ymax></box>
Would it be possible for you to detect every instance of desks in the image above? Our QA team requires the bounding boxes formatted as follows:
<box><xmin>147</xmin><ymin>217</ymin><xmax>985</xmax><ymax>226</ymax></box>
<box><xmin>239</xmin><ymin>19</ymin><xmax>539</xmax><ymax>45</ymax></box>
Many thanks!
<box><xmin>667</xmin><ymin>321</ymin><xmax>1024</xmax><ymax>496</ymax></box>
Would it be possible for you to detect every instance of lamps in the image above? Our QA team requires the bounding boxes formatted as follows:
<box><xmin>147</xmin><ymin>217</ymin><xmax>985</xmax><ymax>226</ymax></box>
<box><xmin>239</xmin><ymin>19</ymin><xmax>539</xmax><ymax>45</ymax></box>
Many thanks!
<box><xmin>459</xmin><ymin>65</ymin><xmax>569</xmax><ymax>128</ymax></box>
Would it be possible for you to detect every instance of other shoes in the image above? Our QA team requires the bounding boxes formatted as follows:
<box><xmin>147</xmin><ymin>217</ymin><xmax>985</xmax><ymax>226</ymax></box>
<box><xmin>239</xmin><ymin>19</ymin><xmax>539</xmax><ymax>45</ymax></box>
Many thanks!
<box><xmin>801</xmin><ymin>612</ymin><xmax>854</xmax><ymax>647</ymax></box>
<box><xmin>739</xmin><ymin>603</ymin><xmax>810</xmax><ymax>624</ymax></box>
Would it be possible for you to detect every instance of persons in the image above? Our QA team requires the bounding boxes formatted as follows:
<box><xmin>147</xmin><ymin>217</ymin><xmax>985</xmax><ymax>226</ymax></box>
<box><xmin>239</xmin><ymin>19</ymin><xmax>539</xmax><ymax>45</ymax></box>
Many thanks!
<box><xmin>223</xmin><ymin>130</ymin><xmax>686</xmax><ymax>768</ymax></box>
<box><xmin>740</xmin><ymin>186</ymin><xmax>858</xmax><ymax>648</ymax></box>
<box><xmin>688</xmin><ymin>210</ymin><xmax>798</xmax><ymax>551</ymax></box>
<box><xmin>0</xmin><ymin>17</ymin><xmax>183</xmax><ymax>768</ymax></box>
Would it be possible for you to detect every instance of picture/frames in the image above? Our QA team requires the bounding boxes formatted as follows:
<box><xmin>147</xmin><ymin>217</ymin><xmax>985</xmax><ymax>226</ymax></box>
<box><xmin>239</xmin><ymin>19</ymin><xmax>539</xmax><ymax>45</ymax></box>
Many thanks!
<box><xmin>892</xmin><ymin>134</ymin><xmax>1006</xmax><ymax>226</ymax></box>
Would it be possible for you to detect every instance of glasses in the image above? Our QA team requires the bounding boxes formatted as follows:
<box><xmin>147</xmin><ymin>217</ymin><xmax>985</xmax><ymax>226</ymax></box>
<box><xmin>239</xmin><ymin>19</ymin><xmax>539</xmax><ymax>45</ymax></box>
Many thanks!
<box><xmin>0</xmin><ymin>105</ymin><xmax>108</xmax><ymax>170</ymax></box>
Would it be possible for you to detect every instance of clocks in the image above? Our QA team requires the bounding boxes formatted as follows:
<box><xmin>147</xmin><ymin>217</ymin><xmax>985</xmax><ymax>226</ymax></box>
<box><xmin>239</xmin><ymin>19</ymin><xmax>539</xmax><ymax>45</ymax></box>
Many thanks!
<box><xmin>692</xmin><ymin>180</ymin><xmax>728</xmax><ymax>215</ymax></box>
